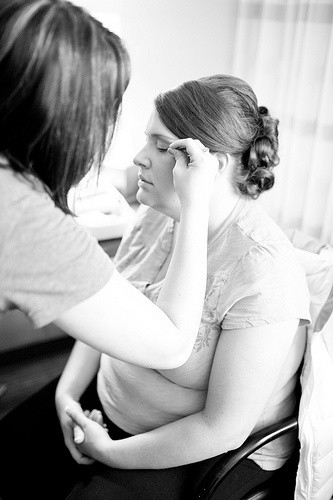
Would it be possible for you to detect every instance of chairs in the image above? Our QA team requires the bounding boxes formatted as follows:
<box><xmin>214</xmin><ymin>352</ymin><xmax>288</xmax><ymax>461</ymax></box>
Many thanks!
<box><xmin>191</xmin><ymin>229</ymin><xmax>333</xmax><ymax>500</ymax></box>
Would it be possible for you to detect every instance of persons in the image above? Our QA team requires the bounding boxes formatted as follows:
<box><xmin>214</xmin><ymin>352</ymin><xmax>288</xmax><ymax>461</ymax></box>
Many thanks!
<box><xmin>0</xmin><ymin>1</ymin><xmax>217</xmax><ymax>368</ymax></box>
<box><xmin>0</xmin><ymin>74</ymin><xmax>313</xmax><ymax>500</ymax></box>
